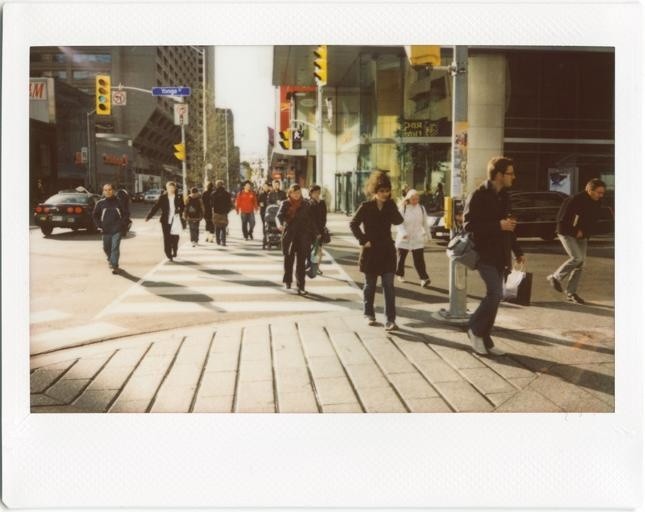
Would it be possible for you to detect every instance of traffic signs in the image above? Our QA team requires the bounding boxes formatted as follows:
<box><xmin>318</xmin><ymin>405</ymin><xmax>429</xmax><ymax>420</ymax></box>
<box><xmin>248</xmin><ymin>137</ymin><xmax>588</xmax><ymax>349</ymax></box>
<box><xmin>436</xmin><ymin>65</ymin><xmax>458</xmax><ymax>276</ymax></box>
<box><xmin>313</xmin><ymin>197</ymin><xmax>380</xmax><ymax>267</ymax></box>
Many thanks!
<box><xmin>151</xmin><ymin>85</ymin><xmax>192</xmax><ymax>97</ymax></box>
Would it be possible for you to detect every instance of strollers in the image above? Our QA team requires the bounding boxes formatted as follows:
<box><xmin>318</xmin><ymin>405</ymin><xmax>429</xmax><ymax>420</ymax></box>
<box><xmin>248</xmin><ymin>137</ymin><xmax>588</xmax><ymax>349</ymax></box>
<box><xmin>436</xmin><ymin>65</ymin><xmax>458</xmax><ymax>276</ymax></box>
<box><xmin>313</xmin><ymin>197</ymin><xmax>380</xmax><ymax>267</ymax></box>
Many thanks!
<box><xmin>258</xmin><ymin>203</ymin><xmax>284</xmax><ymax>249</ymax></box>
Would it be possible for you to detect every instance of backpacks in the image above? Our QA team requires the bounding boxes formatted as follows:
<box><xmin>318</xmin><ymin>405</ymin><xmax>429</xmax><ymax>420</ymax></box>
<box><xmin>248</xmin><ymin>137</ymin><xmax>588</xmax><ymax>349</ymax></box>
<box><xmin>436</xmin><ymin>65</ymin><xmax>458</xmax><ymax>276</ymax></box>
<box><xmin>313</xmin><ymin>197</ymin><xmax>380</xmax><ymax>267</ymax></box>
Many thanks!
<box><xmin>187</xmin><ymin>196</ymin><xmax>201</xmax><ymax>218</ymax></box>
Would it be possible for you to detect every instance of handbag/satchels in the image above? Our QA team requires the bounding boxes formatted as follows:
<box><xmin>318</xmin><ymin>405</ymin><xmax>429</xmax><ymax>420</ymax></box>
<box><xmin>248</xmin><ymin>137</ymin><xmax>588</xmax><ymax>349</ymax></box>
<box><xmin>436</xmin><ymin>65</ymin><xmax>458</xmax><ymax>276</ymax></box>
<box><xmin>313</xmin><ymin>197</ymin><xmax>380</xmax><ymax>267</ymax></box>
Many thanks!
<box><xmin>322</xmin><ymin>228</ymin><xmax>330</xmax><ymax>243</ymax></box>
<box><xmin>447</xmin><ymin>231</ymin><xmax>482</xmax><ymax>271</ymax></box>
<box><xmin>304</xmin><ymin>239</ymin><xmax>323</xmax><ymax>278</ymax></box>
<box><xmin>212</xmin><ymin>213</ymin><xmax>228</xmax><ymax>226</ymax></box>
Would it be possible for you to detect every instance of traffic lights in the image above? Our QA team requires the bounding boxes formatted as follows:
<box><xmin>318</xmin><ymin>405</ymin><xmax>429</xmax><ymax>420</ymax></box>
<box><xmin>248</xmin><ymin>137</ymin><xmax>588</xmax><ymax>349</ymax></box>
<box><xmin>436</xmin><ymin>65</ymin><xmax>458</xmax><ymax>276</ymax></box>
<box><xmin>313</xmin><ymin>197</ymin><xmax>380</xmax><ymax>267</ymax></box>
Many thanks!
<box><xmin>292</xmin><ymin>129</ymin><xmax>303</xmax><ymax>149</ymax></box>
<box><xmin>279</xmin><ymin>128</ymin><xmax>291</xmax><ymax>151</ymax></box>
<box><xmin>313</xmin><ymin>45</ymin><xmax>328</xmax><ymax>87</ymax></box>
<box><xmin>172</xmin><ymin>142</ymin><xmax>186</xmax><ymax>162</ymax></box>
<box><xmin>95</xmin><ymin>74</ymin><xmax>111</xmax><ymax>115</ymax></box>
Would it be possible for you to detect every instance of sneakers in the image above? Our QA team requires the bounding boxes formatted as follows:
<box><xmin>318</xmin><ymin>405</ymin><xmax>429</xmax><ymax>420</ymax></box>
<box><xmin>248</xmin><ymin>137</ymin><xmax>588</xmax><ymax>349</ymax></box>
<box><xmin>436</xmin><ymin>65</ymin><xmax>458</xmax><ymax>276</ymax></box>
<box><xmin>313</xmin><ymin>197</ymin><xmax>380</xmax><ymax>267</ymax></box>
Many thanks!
<box><xmin>365</xmin><ymin>315</ymin><xmax>377</xmax><ymax>325</ymax></box>
<box><xmin>546</xmin><ymin>274</ymin><xmax>563</xmax><ymax>293</ymax></box>
<box><xmin>420</xmin><ymin>279</ymin><xmax>430</xmax><ymax>288</ymax></box>
<box><xmin>298</xmin><ymin>289</ymin><xmax>307</xmax><ymax>295</ymax></box>
<box><xmin>567</xmin><ymin>293</ymin><xmax>584</xmax><ymax>305</ymax></box>
<box><xmin>395</xmin><ymin>275</ymin><xmax>407</xmax><ymax>283</ymax></box>
<box><xmin>384</xmin><ymin>320</ymin><xmax>399</xmax><ymax>331</ymax></box>
<box><xmin>467</xmin><ymin>328</ymin><xmax>494</xmax><ymax>355</ymax></box>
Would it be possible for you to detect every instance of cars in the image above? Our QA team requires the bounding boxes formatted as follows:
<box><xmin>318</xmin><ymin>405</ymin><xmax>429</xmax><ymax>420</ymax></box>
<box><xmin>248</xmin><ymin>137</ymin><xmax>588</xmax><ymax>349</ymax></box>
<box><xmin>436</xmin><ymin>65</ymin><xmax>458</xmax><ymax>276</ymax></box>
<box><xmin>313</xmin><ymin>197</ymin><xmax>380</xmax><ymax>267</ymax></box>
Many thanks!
<box><xmin>33</xmin><ymin>187</ymin><xmax>102</xmax><ymax>237</ymax></box>
<box><xmin>131</xmin><ymin>187</ymin><xmax>205</xmax><ymax>204</ymax></box>
<box><xmin>430</xmin><ymin>190</ymin><xmax>612</xmax><ymax>248</ymax></box>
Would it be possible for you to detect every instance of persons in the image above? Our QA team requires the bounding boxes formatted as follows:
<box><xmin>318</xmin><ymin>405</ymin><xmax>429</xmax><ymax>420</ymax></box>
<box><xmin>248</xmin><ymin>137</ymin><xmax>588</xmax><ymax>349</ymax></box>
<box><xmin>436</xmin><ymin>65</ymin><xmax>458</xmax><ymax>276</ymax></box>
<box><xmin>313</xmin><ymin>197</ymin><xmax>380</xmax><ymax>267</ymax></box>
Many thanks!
<box><xmin>463</xmin><ymin>157</ymin><xmax>526</xmax><ymax>356</ymax></box>
<box><xmin>350</xmin><ymin>173</ymin><xmax>404</xmax><ymax>331</ymax></box>
<box><xmin>547</xmin><ymin>178</ymin><xmax>608</xmax><ymax>304</ymax></box>
<box><xmin>92</xmin><ymin>183</ymin><xmax>128</xmax><ymax>274</ymax></box>
<box><xmin>144</xmin><ymin>174</ymin><xmax>327</xmax><ymax>297</ymax></box>
<box><xmin>395</xmin><ymin>188</ymin><xmax>433</xmax><ymax>287</ymax></box>
